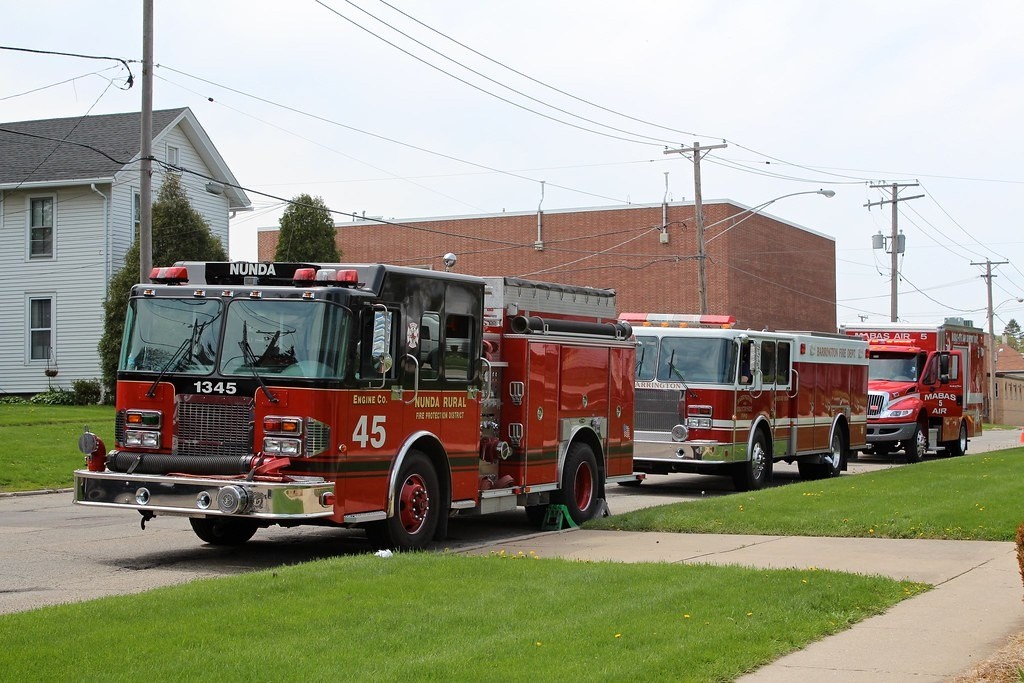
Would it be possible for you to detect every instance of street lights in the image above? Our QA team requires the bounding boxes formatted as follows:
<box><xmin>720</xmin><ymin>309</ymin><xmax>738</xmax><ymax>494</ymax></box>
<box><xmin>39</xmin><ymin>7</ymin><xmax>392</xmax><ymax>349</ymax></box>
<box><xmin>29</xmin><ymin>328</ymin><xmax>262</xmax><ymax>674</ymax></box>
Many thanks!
<box><xmin>694</xmin><ymin>187</ymin><xmax>838</xmax><ymax>314</ymax></box>
<box><xmin>986</xmin><ymin>298</ymin><xmax>1024</xmax><ymax>424</ymax></box>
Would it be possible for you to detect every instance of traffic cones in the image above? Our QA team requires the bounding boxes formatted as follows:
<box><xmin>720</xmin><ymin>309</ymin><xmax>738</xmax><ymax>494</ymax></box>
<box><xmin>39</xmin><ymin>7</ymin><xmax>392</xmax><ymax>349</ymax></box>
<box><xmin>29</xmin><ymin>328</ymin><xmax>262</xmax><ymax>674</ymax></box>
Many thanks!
<box><xmin>1018</xmin><ymin>428</ymin><xmax>1024</xmax><ymax>445</ymax></box>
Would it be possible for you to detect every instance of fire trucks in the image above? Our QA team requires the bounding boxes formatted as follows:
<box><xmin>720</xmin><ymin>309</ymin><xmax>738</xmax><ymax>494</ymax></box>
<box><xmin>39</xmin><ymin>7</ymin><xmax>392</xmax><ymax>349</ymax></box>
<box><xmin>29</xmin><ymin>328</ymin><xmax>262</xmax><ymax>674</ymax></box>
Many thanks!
<box><xmin>837</xmin><ymin>316</ymin><xmax>983</xmax><ymax>463</ymax></box>
<box><xmin>616</xmin><ymin>311</ymin><xmax>870</xmax><ymax>492</ymax></box>
<box><xmin>70</xmin><ymin>253</ymin><xmax>644</xmax><ymax>552</ymax></box>
